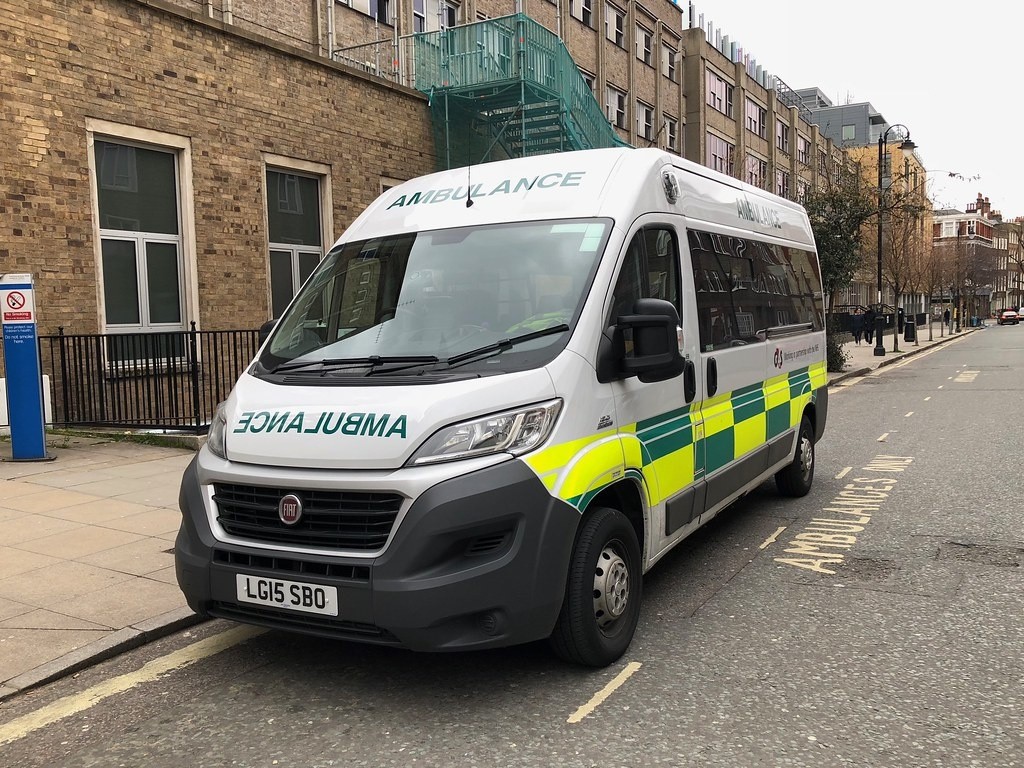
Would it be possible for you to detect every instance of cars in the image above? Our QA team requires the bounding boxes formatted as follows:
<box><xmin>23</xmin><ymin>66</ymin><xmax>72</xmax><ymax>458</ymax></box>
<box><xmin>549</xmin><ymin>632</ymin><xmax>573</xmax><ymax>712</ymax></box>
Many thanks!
<box><xmin>996</xmin><ymin>310</ymin><xmax>1020</xmax><ymax>325</ymax></box>
<box><xmin>1018</xmin><ymin>308</ymin><xmax>1024</xmax><ymax>320</ymax></box>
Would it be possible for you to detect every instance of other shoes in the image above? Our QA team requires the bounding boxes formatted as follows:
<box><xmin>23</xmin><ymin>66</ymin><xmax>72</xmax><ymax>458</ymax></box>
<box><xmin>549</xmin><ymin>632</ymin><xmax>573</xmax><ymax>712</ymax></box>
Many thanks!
<box><xmin>855</xmin><ymin>344</ymin><xmax>859</xmax><ymax>347</ymax></box>
<box><xmin>869</xmin><ymin>343</ymin><xmax>872</xmax><ymax>346</ymax></box>
<box><xmin>864</xmin><ymin>342</ymin><xmax>868</xmax><ymax>346</ymax></box>
<box><xmin>859</xmin><ymin>343</ymin><xmax>861</xmax><ymax>347</ymax></box>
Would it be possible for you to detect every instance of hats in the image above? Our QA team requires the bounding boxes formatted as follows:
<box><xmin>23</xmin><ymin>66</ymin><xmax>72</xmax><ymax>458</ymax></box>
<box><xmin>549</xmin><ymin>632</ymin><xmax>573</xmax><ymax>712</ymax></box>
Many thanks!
<box><xmin>867</xmin><ymin>305</ymin><xmax>872</xmax><ymax>310</ymax></box>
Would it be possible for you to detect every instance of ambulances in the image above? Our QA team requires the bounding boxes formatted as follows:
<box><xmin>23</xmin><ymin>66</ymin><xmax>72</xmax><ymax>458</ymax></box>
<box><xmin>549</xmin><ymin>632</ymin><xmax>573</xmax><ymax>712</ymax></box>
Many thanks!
<box><xmin>173</xmin><ymin>147</ymin><xmax>829</xmax><ymax>675</ymax></box>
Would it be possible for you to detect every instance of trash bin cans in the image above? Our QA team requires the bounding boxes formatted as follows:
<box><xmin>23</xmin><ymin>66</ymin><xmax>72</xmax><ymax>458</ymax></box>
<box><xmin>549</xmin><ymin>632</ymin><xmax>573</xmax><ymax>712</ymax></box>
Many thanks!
<box><xmin>904</xmin><ymin>322</ymin><xmax>916</xmax><ymax>342</ymax></box>
<box><xmin>970</xmin><ymin>316</ymin><xmax>981</xmax><ymax>326</ymax></box>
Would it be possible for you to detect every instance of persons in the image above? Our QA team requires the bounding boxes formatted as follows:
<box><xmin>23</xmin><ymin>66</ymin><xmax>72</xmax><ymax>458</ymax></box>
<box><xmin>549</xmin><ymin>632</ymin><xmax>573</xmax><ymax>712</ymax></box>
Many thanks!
<box><xmin>953</xmin><ymin>305</ymin><xmax>968</xmax><ymax>326</ymax></box>
<box><xmin>944</xmin><ymin>309</ymin><xmax>951</xmax><ymax>327</ymax></box>
<box><xmin>1011</xmin><ymin>307</ymin><xmax>1020</xmax><ymax>312</ymax></box>
<box><xmin>864</xmin><ymin>304</ymin><xmax>878</xmax><ymax>347</ymax></box>
<box><xmin>850</xmin><ymin>307</ymin><xmax>865</xmax><ymax>347</ymax></box>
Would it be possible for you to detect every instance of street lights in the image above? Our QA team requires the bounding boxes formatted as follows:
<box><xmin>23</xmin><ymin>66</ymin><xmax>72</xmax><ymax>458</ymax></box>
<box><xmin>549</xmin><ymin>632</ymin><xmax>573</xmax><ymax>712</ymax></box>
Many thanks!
<box><xmin>955</xmin><ymin>224</ymin><xmax>977</xmax><ymax>334</ymax></box>
<box><xmin>870</xmin><ymin>123</ymin><xmax>919</xmax><ymax>357</ymax></box>
